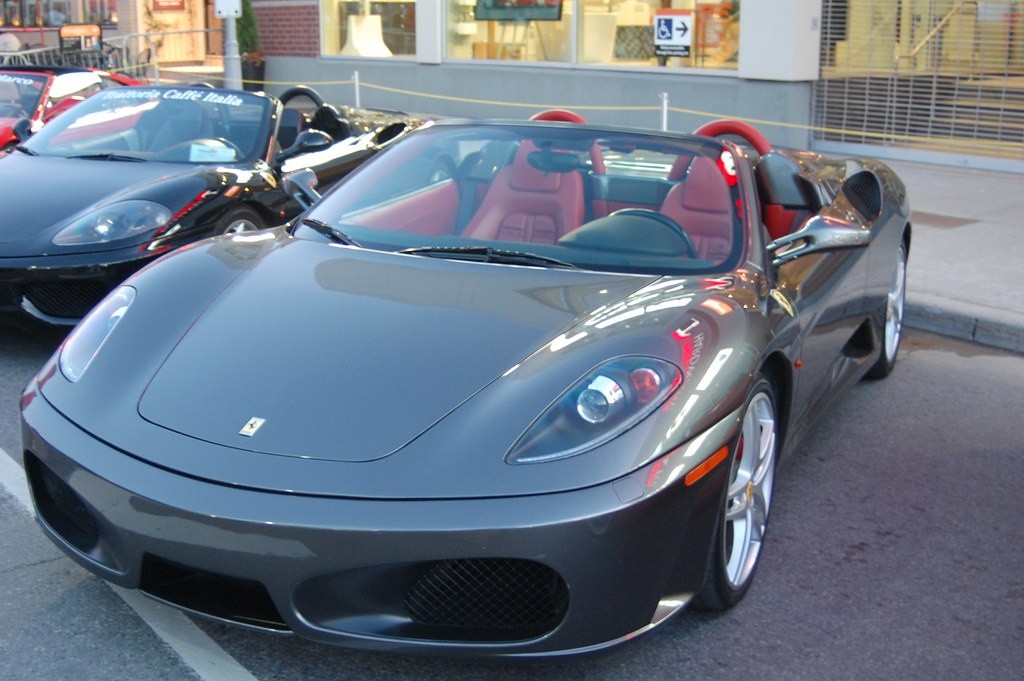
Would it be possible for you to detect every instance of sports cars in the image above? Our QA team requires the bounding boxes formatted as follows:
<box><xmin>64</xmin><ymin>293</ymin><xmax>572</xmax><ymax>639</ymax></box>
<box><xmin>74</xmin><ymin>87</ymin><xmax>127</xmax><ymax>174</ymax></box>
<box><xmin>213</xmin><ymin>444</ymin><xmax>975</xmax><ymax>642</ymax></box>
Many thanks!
<box><xmin>0</xmin><ymin>63</ymin><xmax>163</xmax><ymax>159</ymax></box>
<box><xmin>1</xmin><ymin>81</ymin><xmax>413</xmax><ymax>327</ymax></box>
<box><xmin>16</xmin><ymin>111</ymin><xmax>913</xmax><ymax>662</ymax></box>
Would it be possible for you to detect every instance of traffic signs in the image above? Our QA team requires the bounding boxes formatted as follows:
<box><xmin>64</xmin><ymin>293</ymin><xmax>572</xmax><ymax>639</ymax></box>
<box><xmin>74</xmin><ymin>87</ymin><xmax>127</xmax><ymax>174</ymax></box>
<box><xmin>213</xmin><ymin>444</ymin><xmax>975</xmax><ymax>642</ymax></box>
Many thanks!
<box><xmin>654</xmin><ymin>13</ymin><xmax>692</xmax><ymax>57</ymax></box>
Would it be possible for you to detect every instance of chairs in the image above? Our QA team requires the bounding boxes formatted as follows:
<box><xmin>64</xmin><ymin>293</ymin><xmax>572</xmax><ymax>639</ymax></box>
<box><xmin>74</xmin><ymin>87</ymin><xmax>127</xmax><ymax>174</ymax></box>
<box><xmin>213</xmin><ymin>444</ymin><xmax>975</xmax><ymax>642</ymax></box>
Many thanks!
<box><xmin>528</xmin><ymin>110</ymin><xmax>608</xmax><ymax>221</ymax></box>
<box><xmin>656</xmin><ymin>155</ymin><xmax>734</xmax><ymax>264</ymax></box>
<box><xmin>145</xmin><ymin>101</ymin><xmax>214</xmax><ymax>153</ymax></box>
<box><xmin>276</xmin><ymin>109</ymin><xmax>303</xmax><ymax>151</ymax></box>
<box><xmin>461</xmin><ymin>140</ymin><xmax>583</xmax><ymax>247</ymax></box>
<box><xmin>279</xmin><ymin>85</ymin><xmax>350</xmax><ymax>139</ymax></box>
<box><xmin>668</xmin><ymin>118</ymin><xmax>796</xmax><ymax>238</ymax></box>
